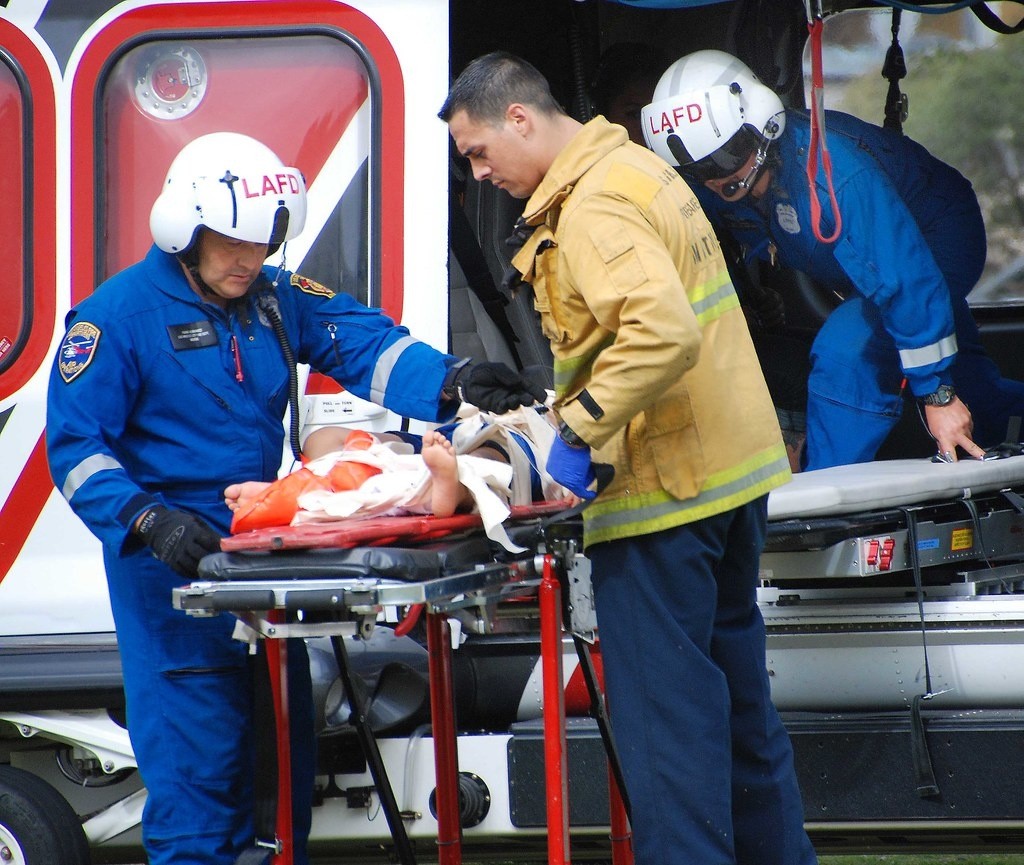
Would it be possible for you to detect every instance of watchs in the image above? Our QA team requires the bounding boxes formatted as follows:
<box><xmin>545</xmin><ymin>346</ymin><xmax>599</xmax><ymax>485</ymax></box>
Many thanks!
<box><xmin>560</xmin><ymin>424</ymin><xmax>586</xmax><ymax>446</ymax></box>
<box><xmin>922</xmin><ymin>385</ymin><xmax>955</xmax><ymax>406</ymax></box>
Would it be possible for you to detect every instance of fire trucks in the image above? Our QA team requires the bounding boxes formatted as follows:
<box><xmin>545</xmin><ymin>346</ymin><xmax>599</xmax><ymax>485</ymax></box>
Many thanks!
<box><xmin>0</xmin><ymin>1</ymin><xmax>1024</xmax><ymax>865</ymax></box>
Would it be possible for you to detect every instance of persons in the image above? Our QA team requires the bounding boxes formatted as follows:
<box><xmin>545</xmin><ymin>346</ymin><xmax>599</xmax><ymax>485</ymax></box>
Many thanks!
<box><xmin>438</xmin><ymin>54</ymin><xmax>817</xmax><ymax>865</ymax></box>
<box><xmin>46</xmin><ymin>131</ymin><xmax>547</xmax><ymax>865</ymax></box>
<box><xmin>224</xmin><ymin>389</ymin><xmax>576</xmax><ymax>525</ymax></box>
<box><xmin>592</xmin><ymin>43</ymin><xmax>832</xmax><ymax>473</ymax></box>
<box><xmin>640</xmin><ymin>49</ymin><xmax>1024</xmax><ymax>472</ymax></box>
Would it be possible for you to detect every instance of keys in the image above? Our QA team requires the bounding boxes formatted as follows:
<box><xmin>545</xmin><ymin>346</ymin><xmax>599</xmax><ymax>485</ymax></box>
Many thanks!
<box><xmin>768</xmin><ymin>243</ymin><xmax>777</xmax><ymax>266</ymax></box>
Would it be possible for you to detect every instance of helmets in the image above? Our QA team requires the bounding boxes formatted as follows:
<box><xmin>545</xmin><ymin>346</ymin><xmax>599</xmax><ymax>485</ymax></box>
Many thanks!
<box><xmin>640</xmin><ymin>49</ymin><xmax>787</xmax><ymax>181</ymax></box>
<box><xmin>150</xmin><ymin>132</ymin><xmax>305</xmax><ymax>254</ymax></box>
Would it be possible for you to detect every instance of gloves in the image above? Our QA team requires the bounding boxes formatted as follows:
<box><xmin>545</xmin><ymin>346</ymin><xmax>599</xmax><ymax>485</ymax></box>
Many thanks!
<box><xmin>444</xmin><ymin>356</ymin><xmax>548</xmax><ymax>415</ymax></box>
<box><xmin>136</xmin><ymin>504</ymin><xmax>223</xmax><ymax>580</ymax></box>
<box><xmin>545</xmin><ymin>434</ymin><xmax>594</xmax><ymax>502</ymax></box>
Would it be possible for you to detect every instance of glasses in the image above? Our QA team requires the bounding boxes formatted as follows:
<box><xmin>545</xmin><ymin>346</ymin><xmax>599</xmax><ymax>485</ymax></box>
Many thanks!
<box><xmin>671</xmin><ymin>127</ymin><xmax>754</xmax><ymax>181</ymax></box>
